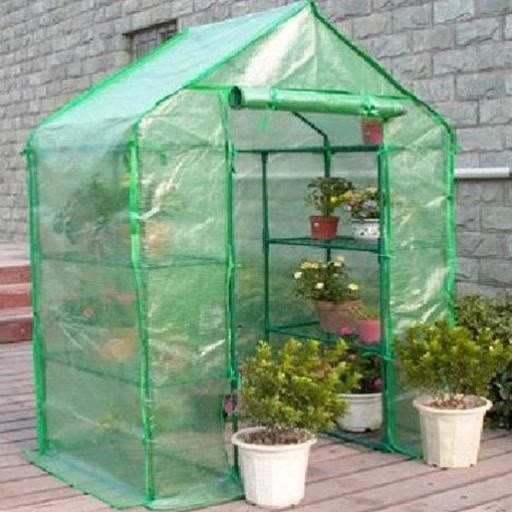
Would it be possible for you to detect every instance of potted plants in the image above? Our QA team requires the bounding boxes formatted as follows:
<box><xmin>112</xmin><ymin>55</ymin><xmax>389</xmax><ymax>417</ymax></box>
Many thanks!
<box><xmin>54</xmin><ymin>172</ymin><xmax>185</xmax><ymax>368</ymax></box>
<box><xmin>304</xmin><ymin>178</ymin><xmax>357</xmax><ymax>240</ymax></box>
<box><xmin>231</xmin><ymin>337</ymin><xmax>363</xmax><ymax>511</ymax></box>
<box><xmin>290</xmin><ymin>259</ymin><xmax>512</xmax><ymax>469</ymax></box>
<box><xmin>340</xmin><ymin>188</ymin><xmax>387</xmax><ymax>242</ymax></box>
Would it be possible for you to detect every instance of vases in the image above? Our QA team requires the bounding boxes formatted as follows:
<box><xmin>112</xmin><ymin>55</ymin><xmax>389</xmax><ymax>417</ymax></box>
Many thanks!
<box><xmin>359</xmin><ymin>115</ymin><xmax>383</xmax><ymax>145</ymax></box>
